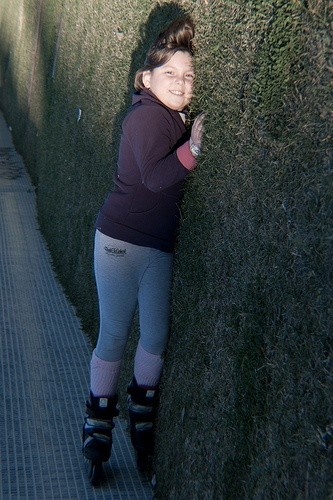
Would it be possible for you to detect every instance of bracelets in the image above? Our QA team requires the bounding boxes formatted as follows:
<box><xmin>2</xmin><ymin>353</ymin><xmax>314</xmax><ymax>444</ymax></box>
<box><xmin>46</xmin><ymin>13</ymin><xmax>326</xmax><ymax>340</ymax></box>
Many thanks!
<box><xmin>189</xmin><ymin>137</ymin><xmax>201</xmax><ymax>155</ymax></box>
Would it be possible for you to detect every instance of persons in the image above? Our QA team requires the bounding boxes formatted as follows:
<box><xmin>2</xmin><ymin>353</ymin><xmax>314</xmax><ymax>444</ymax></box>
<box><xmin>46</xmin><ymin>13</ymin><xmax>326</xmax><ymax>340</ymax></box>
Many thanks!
<box><xmin>83</xmin><ymin>21</ymin><xmax>207</xmax><ymax>485</ymax></box>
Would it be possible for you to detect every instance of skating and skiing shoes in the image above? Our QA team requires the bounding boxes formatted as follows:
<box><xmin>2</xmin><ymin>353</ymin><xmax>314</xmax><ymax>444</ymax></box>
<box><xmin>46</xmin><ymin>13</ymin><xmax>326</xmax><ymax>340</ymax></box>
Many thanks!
<box><xmin>82</xmin><ymin>391</ymin><xmax>120</xmax><ymax>484</ymax></box>
<box><xmin>124</xmin><ymin>375</ymin><xmax>162</xmax><ymax>469</ymax></box>
